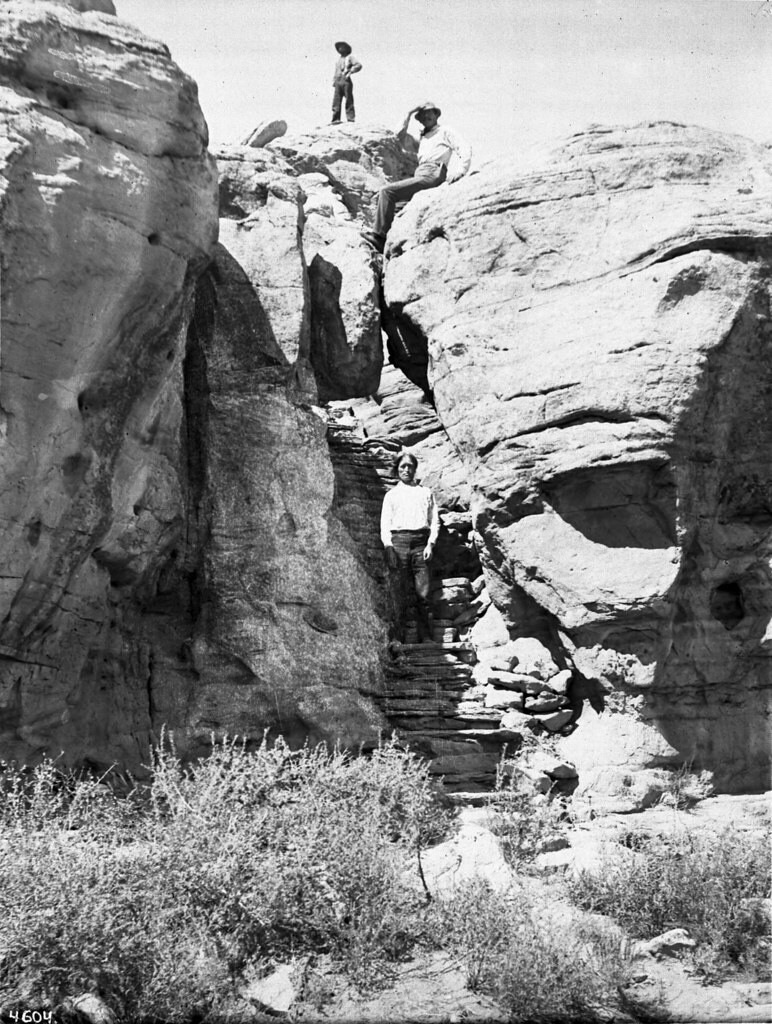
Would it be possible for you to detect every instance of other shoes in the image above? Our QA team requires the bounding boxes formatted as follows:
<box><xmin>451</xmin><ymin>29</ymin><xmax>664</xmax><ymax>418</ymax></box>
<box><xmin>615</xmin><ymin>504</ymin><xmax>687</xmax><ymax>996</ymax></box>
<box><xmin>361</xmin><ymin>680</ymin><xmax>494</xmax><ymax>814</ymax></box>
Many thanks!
<box><xmin>360</xmin><ymin>231</ymin><xmax>385</xmax><ymax>251</ymax></box>
<box><xmin>421</xmin><ymin>636</ymin><xmax>434</xmax><ymax>643</ymax></box>
<box><xmin>396</xmin><ymin>638</ymin><xmax>405</xmax><ymax>644</ymax></box>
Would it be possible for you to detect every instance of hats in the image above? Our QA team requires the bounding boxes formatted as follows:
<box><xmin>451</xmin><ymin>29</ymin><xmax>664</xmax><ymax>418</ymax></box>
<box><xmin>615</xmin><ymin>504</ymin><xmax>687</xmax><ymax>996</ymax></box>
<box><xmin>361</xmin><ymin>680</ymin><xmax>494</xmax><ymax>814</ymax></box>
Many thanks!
<box><xmin>415</xmin><ymin>102</ymin><xmax>441</xmax><ymax>120</ymax></box>
<box><xmin>335</xmin><ymin>42</ymin><xmax>351</xmax><ymax>55</ymax></box>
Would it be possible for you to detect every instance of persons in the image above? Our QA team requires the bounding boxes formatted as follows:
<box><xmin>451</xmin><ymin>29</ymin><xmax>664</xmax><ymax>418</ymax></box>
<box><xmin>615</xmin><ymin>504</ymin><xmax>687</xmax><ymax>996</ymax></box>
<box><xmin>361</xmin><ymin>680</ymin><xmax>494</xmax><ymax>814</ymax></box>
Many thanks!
<box><xmin>327</xmin><ymin>40</ymin><xmax>363</xmax><ymax>126</ymax></box>
<box><xmin>360</xmin><ymin>101</ymin><xmax>473</xmax><ymax>257</ymax></box>
<box><xmin>378</xmin><ymin>451</ymin><xmax>442</xmax><ymax>645</ymax></box>
